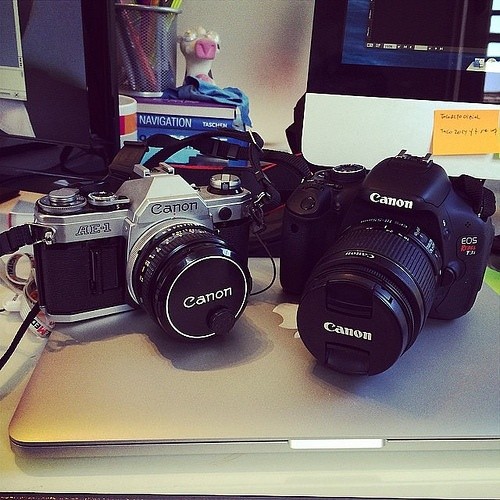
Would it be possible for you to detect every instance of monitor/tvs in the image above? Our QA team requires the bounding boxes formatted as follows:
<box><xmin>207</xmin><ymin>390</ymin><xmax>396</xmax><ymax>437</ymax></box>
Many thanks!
<box><xmin>301</xmin><ymin>0</ymin><xmax>499</xmax><ymax>181</ymax></box>
<box><xmin>1</xmin><ymin>0</ymin><xmax>119</xmax><ymax>151</ymax></box>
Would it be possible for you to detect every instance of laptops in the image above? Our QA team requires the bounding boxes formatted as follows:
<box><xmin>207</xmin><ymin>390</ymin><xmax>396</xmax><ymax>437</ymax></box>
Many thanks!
<box><xmin>7</xmin><ymin>258</ymin><xmax>500</xmax><ymax>459</ymax></box>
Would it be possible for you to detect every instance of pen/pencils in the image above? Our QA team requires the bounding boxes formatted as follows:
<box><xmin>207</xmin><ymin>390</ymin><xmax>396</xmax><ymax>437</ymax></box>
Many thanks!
<box><xmin>110</xmin><ymin>0</ymin><xmax>186</xmax><ymax>95</ymax></box>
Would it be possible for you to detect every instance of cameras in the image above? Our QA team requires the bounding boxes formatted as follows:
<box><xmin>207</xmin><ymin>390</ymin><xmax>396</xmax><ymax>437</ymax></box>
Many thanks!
<box><xmin>32</xmin><ymin>170</ymin><xmax>272</xmax><ymax>346</ymax></box>
<box><xmin>279</xmin><ymin>154</ymin><xmax>496</xmax><ymax>378</ymax></box>
<box><xmin>185</xmin><ymin>153</ymin><xmax>301</xmax><ymax>261</ymax></box>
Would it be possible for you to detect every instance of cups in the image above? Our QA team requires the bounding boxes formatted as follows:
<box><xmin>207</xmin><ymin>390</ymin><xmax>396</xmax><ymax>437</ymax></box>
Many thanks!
<box><xmin>118</xmin><ymin>95</ymin><xmax>138</xmax><ymax>150</ymax></box>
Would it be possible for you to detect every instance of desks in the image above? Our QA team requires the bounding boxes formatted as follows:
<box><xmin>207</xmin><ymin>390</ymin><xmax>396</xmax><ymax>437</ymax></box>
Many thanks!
<box><xmin>0</xmin><ymin>141</ymin><xmax>500</xmax><ymax>498</ymax></box>
